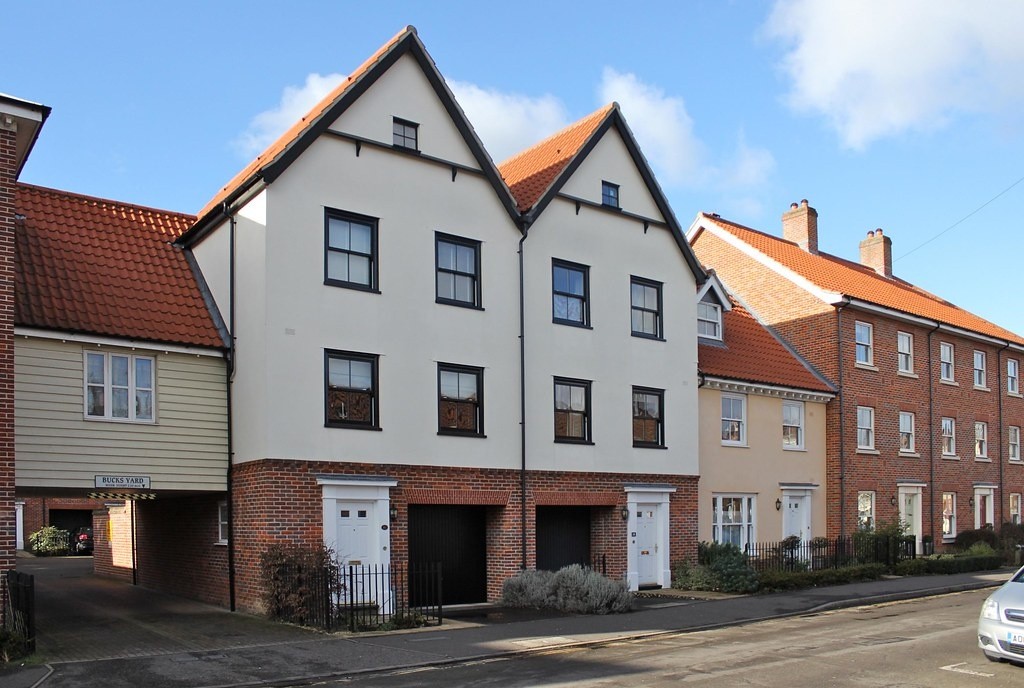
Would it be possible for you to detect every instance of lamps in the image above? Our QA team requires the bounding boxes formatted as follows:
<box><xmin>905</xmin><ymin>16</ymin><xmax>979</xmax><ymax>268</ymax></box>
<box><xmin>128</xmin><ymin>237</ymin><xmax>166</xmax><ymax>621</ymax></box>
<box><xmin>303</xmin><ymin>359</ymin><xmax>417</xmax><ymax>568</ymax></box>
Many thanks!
<box><xmin>776</xmin><ymin>498</ymin><xmax>782</xmax><ymax>511</ymax></box>
<box><xmin>621</xmin><ymin>506</ymin><xmax>629</xmax><ymax>520</ymax></box>
<box><xmin>969</xmin><ymin>497</ymin><xmax>974</xmax><ymax>506</ymax></box>
<box><xmin>891</xmin><ymin>496</ymin><xmax>896</xmax><ymax>505</ymax></box>
<box><xmin>389</xmin><ymin>504</ymin><xmax>398</xmax><ymax>522</ymax></box>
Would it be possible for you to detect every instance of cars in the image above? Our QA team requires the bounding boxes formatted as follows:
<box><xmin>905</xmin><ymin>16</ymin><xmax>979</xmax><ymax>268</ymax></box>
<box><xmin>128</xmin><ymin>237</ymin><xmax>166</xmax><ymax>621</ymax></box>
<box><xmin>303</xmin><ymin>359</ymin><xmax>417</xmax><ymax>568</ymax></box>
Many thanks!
<box><xmin>68</xmin><ymin>526</ymin><xmax>94</xmax><ymax>554</ymax></box>
<box><xmin>977</xmin><ymin>564</ymin><xmax>1024</xmax><ymax>665</ymax></box>
